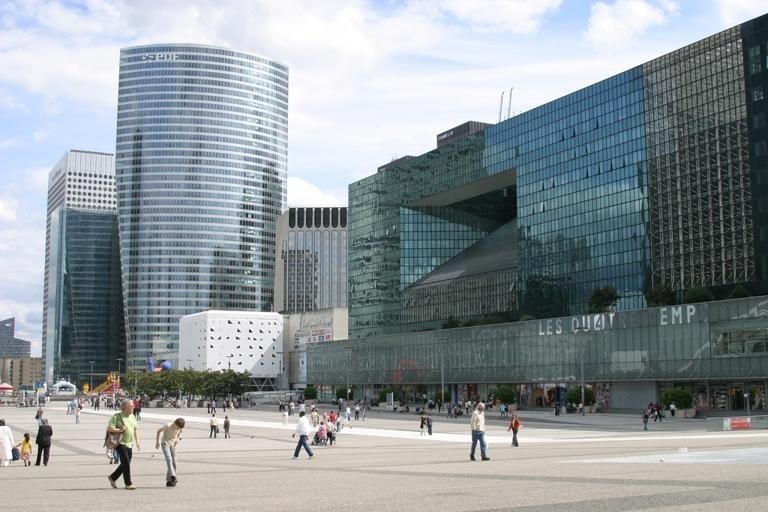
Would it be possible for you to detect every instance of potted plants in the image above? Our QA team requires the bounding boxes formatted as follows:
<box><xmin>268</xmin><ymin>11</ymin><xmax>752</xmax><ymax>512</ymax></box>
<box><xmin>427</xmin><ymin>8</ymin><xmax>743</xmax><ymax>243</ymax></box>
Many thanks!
<box><xmin>565</xmin><ymin>385</ymin><xmax>597</xmax><ymax>414</ymax></box>
<box><xmin>336</xmin><ymin>386</ymin><xmax>355</xmax><ymax>404</ymax></box>
<box><xmin>380</xmin><ymin>387</ymin><xmax>403</xmax><ymax>407</ymax></box>
<box><xmin>488</xmin><ymin>385</ymin><xmax>518</xmax><ymax>412</ymax></box>
<box><xmin>303</xmin><ymin>385</ymin><xmax>320</xmax><ymax>405</ymax></box>
<box><xmin>427</xmin><ymin>389</ymin><xmax>452</xmax><ymax>412</ymax></box>
<box><xmin>657</xmin><ymin>387</ymin><xmax>697</xmax><ymax>419</ymax></box>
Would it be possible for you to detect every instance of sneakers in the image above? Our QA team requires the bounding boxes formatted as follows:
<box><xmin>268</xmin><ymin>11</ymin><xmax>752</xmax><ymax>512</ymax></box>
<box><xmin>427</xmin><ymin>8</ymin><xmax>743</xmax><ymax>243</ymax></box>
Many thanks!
<box><xmin>165</xmin><ymin>481</ymin><xmax>176</xmax><ymax>487</ymax></box>
<box><xmin>171</xmin><ymin>476</ymin><xmax>178</xmax><ymax>484</ymax></box>
<box><xmin>482</xmin><ymin>457</ymin><xmax>490</xmax><ymax>461</ymax></box>
<box><xmin>125</xmin><ymin>483</ymin><xmax>135</xmax><ymax>489</ymax></box>
<box><xmin>108</xmin><ymin>475</ymin><xmax>118</xmax><ymax>489</ymax></box>
<box><xmin>470</xmin><ymin>453</ymin><xmax>476</xmax><ymax>461</ymax></box>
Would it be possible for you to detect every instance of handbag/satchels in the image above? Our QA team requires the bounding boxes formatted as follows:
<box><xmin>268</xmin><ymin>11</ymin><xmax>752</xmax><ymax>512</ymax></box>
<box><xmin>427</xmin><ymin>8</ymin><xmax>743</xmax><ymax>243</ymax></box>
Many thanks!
<box><xmin>104</xmin><ymin>414</ymin><xmax>123</xmax><ymax>449</ymax></box>
<box><xmin>11</xmin><ymin>447</ymin><xmax>20</xmax><ymax>461</ymax></box>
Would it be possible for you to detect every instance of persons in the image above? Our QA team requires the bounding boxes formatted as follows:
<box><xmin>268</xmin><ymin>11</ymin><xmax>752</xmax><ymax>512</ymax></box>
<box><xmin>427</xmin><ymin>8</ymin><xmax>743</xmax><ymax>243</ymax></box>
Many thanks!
<box><xmin>579</xmin><ymin>402</ymin><xmax>583</xmax><ymax>412</ymax></box>
<box><xmin>470</xmin><ymin>401</ymin><xmax>490</xmax><ymax>461</ymax></box>
<box><xmin>556</xmin><ymin>401</ymin><xmax>560</xmax><ymax>416</ymax></box>
<box><xmin>642</xmin><ymin>400</ymin><xmax>676</xmax><ymax>430</ymax></box>
<box><xmin>107</xmin><ymin>398</ymin><xmax>141</xmax><ymax>491</ymax></box>
<box><xmin>156</xmin><ymin>418</ymin><xmax>185</xmax><ymax>486</ymax></box>
<box><xmin>291</xmin><ymin>411</ymin><xmax>316</xmax><ymax>460</ymax></box>
<box><xmin>508</xmin><ymin>414</ymin><xmax>520</xmax><ymax>449</ymax></box>
<box><xmin>276</xmin><ymin>392</ymin><xmax>509</xmax><ymax>445</ymax></box>
<box><xmin>0</xmin><ymin>389</ymin><xmax>257</xmax><ymax>466</ymax></box>
